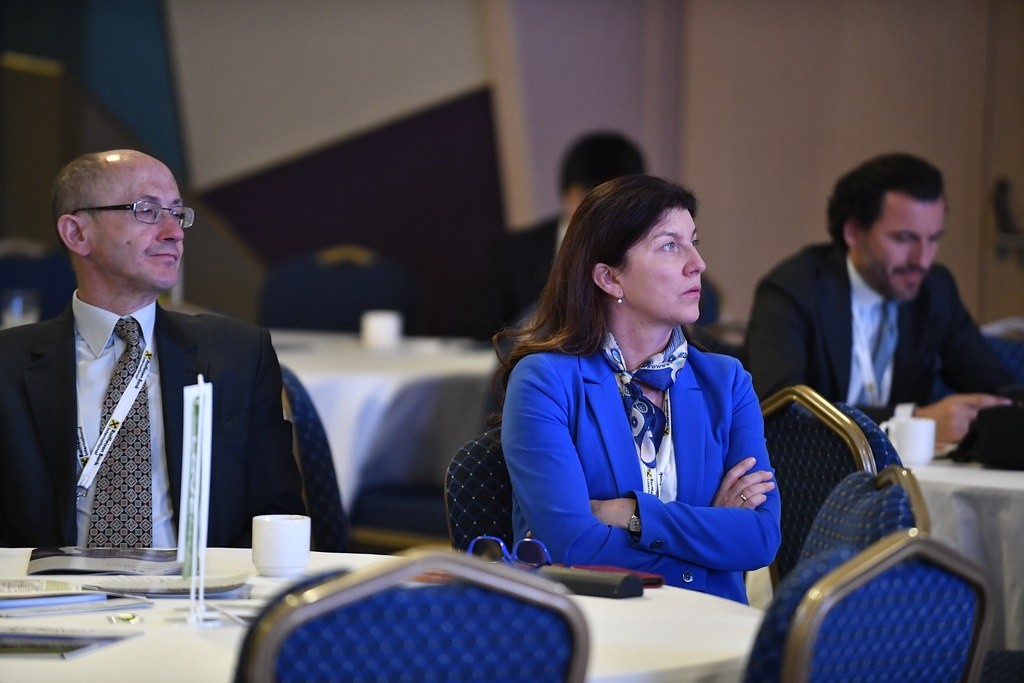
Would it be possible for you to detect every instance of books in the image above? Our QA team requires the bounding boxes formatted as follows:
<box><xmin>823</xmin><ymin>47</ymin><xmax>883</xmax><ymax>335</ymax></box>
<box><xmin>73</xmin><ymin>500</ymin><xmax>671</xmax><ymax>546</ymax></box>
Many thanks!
<box><xmin>27</xmin><ymin>548</ymin><xmax>200</xmax><ymax>576</ymax></box>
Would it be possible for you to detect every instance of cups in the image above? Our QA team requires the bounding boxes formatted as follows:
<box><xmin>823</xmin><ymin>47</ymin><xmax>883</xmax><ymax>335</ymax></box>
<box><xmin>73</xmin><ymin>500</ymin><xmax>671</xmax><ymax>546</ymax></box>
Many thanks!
<box><xmin>879</xmin><ymin>418</ymin><xmax>935</xmax><ymax>470</ymax></box>
<box><xmin>252</xmin><ymin>514</ymin><xmax>311</xmax><ymax>577</ymax></box>
<box><xmin>361</xmin><ymin>310</ymin><xmax>402</xmax><ymax>349</ymax></box>
<box><xmin>0</xmin><ymin>547</ymin><xmax>37</xmax><ymax>579</ymax></box>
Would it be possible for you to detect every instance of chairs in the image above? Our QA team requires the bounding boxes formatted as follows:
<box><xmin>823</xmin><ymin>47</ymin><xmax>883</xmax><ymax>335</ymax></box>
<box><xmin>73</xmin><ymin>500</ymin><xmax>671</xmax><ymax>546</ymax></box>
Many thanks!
<box><xmin>232</xmin><ymin>338</ymin><xmax>1024</xmax><ymax>683</ymax></box>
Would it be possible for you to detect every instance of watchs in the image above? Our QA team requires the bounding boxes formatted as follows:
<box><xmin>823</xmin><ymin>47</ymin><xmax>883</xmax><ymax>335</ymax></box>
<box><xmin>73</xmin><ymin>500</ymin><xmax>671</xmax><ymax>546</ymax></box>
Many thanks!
<box><xmin>628</xmin><ymin>497</ymin><xmax>642</xmax><ymax>538</ymax></box>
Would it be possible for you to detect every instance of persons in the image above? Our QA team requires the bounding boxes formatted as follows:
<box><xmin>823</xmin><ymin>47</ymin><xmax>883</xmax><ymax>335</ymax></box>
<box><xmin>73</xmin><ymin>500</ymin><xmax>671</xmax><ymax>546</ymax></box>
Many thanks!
<box><xmin>742</xmin><ymin>152</ymin><xmax>1024</xmax><ymax>469</ymax></box>
<box><xmin>0</xmin><ymin>149</ymin><xmax>314</xmax><ymax>548</ymax></box>
<box><xmin>471</xmin><ymin>132</ymin><xmax>722</xmax><ymax>341</ymax></box>
<box><xmin>485</xmin><ymin>172</ymin><xmax>783</xmax><ymax>606</ymax></box>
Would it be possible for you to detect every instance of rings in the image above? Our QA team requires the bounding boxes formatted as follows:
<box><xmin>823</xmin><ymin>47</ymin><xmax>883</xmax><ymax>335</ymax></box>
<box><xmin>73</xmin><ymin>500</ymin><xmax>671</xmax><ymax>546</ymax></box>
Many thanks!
<box><xmin>739</xmin><ymin>493</ymin><xmax>747</xmax><ymax>502</ymax></box>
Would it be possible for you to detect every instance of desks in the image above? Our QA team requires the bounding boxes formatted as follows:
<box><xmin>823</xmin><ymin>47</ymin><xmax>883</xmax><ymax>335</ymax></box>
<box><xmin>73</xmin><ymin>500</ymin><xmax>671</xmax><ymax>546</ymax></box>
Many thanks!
<box><xmin>0</xmin><ymin>548</ymin><xmax>766</xmax><ymax>683</ymax></box>
<box><xmin>906</xmin><ymin>463</ymin><xmax>1023</xmax><ymax>653</ymax></box>
<box><xmin>267</xmin><ymin>331</ymin><xmax>502</xmax><ymax>520</ymax></box>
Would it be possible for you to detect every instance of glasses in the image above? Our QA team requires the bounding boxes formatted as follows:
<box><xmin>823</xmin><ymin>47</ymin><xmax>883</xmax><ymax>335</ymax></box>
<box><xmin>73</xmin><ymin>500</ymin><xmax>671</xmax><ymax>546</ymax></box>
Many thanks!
<box><xmin>467</xmin><ymin>537</ymin><xmax>550</xmax><ymax>574</ymax></box>
<box><xmin>70</xmin><ymin>200</ymin><xmax>194</xmax><ymax>228</ymax></box>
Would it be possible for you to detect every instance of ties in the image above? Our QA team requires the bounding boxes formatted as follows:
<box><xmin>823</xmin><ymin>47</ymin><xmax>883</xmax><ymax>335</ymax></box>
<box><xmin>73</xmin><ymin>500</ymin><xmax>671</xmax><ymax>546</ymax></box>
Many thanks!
<box><xmin>854</xmin><ymin>299</ymin><xmax>900</xmax><ymax>410</ymax></box>
<box><xmin>87</xmin><ymin>317</ymin><xmax>153</xmax><ymax>551</ymax></box>
